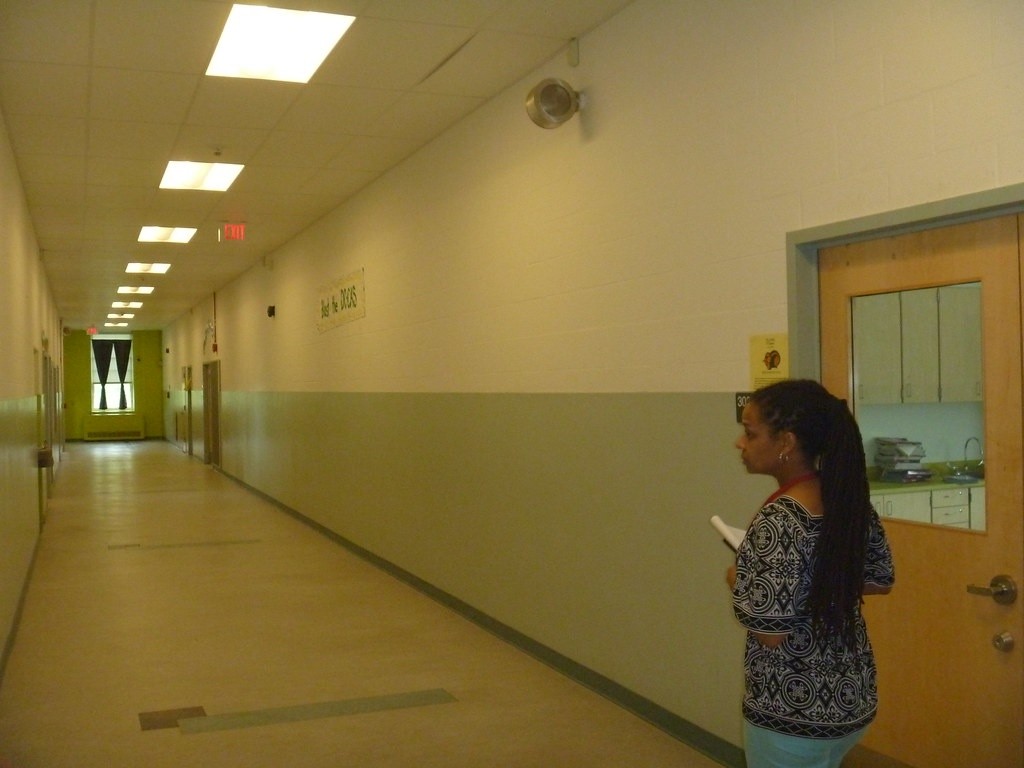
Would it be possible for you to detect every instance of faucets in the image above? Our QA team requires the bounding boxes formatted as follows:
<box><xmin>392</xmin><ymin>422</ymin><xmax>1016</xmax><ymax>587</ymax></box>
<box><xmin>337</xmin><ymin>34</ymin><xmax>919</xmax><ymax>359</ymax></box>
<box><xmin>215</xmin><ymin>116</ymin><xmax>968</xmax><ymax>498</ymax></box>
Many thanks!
<box><xmin>964</xmin><ymin>436</ymin><xmax>984</xmax><ymax>473</ymax></box>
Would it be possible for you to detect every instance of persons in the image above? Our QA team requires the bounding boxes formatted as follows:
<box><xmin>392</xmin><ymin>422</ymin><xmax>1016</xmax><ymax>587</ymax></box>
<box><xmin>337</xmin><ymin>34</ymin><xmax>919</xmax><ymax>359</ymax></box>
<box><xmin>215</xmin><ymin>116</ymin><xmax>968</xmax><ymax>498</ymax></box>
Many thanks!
<box><xmin>725</xmin><ymin>378</ymin><xmax>896</xmax><ymax>768</ymax></box>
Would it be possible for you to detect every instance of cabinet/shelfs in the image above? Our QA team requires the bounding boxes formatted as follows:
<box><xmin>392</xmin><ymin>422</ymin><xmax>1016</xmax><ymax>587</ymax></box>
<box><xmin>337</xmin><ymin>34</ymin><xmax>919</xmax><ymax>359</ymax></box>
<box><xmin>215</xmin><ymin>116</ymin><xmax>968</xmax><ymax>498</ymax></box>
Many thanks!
<box><xmin>852</xmin><ymin>281</ymin><xmax>986</xmax><ymax>531</ymax></box>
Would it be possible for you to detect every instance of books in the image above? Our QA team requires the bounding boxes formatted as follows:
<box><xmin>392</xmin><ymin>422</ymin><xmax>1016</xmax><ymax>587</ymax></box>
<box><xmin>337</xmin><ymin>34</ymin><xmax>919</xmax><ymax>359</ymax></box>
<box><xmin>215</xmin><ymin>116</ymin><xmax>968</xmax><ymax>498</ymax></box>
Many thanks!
<box><xmin>709</xmin><ymin>515</ymin><xmax>748</xmax><ymax>554</ymax></box>
